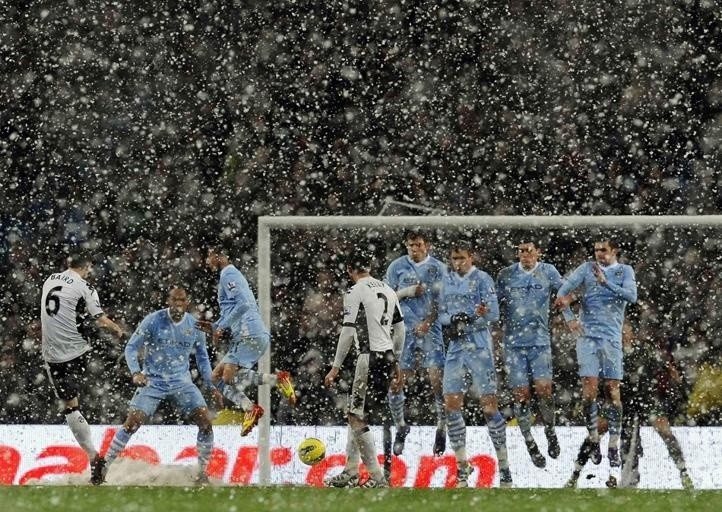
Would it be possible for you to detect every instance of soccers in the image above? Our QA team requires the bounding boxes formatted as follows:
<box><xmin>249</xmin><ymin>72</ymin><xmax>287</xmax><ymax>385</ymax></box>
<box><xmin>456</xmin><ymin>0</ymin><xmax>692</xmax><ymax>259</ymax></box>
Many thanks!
<box><xmin>299</xmin><ymin>437</ymin><xmax>326</xmax><ymax>465</ymax></box>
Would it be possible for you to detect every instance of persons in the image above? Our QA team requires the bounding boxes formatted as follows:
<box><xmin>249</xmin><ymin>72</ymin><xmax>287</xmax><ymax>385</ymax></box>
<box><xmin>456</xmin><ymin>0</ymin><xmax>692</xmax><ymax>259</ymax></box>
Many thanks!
<box><xmin>493</xmin><ymin>236</ymin><xmax>586</xmax><ymax>467</ymax></box>
<box><xmin>384</xmin><ymin>231</ymin><xmax>449</xmax><ymax>455</ymax></box>
<box><xmin>100</xmin><ymin>286</ymin><xmax>225</xmax><ymax>487</ymax></box>
<box><xmin>40</xmin><ymin>249</ymin><xmax>122</xmax><ymax>485</ymax></box>
<box><xmin>277</xmin><ymin>270</ymin><xmax>345</xmax><ymax>424</ymax></box>
<box><xmin>323</xmin><ymin>249</ymin><xmax>406</xmax><ymax>487</ymax></box>
<box><xmin>556</xmin><ymin>234</ymin><xmax>639</xmax><ymax>467</ymax></box>
<box><xmin>195</xmin><ymin>239</ymin><xmax>296</xmax><ymax>436</ymax></box>
<box><xmin>435</xmin><ymin>242</ymin><xmax>513</xmax><ymax>492</ymax></box>
<box><xmin>562</xmin><ymin>319</ymin><xmax>722</xmax><ymax>494</ymax></box>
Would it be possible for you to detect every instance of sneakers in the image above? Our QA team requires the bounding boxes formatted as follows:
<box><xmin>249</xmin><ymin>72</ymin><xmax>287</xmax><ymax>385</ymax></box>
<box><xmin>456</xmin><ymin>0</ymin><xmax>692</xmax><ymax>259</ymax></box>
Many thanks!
<box><xmin>91</xmin><ymin>455</ymin><xmax>109</xmax><ymax>484</ymax></box>
<box><xmin>197</xmin><ymin>470</ymin><xmax>212</xmax><ymax>489</ymax></box>
<box><xmin>433</xmin><ymin>430</ymin><xmax>447</xmax><ymax>457</ymax></box>
<box><xmin>591</xmin><ymin>442</ymin><xmax>640</xmax><ymax>490</ymax></box>
<box><xmin>324</xmin><ymin>471</ymin><xmax>359</xmax><ymax>488</ymax></box>
<box><xmin>562</xmin><ymin>479</ymin><xmax>578</xmax><ymax>490</ymax></box>
<box><xmin>500</xmin><ymin>465</ymin><xmax>513</xmax><ymax>488</ymax></box>
<box><xmin>526</xmin><ymin>439</ymin><xmax>546</xmax><ymax>468</ymax></box>
<box><xmin>454</xmin><ymin>462</ymin><xmax>474</xmax><ymax>489</ymax></box>
<box><xmin>392</xmin><ymin>427</ymin><xmax>410</xmax><ymax>455</ymax></box>
<box><xmin>546</xmin><ymin>431</ymin><xmax>559</xmax><ymax>460</ymax></box>
<box><xmin>240</xmin><ymin>404</ymin><xmax>264</xmax><ymax>436</ymax></box>
<box><xmin>683</xmin><ymin>473</ymin><xmax>697</xmax><ymax>497</ymax></box>
<box><xmin>277</xmin><ymin>371</ymin><xmax>298</xmax><ymax>404</ymax></box>
<box><xmin>360</xmin><ymin>478</ymin><xmax>389</xmax><ymax>488</ymax></box>
